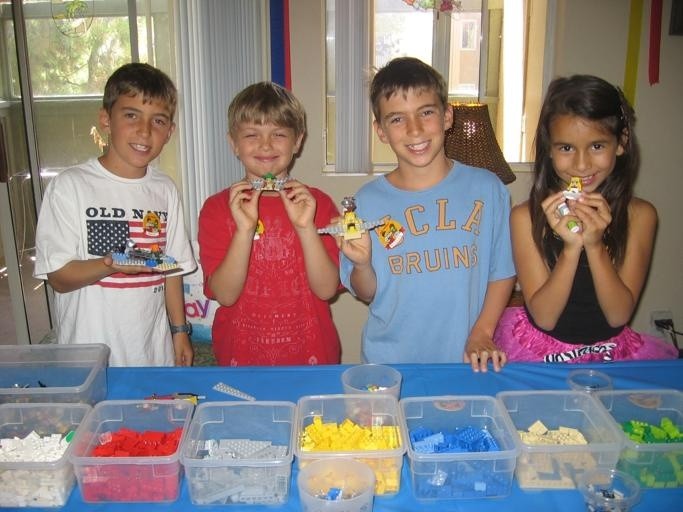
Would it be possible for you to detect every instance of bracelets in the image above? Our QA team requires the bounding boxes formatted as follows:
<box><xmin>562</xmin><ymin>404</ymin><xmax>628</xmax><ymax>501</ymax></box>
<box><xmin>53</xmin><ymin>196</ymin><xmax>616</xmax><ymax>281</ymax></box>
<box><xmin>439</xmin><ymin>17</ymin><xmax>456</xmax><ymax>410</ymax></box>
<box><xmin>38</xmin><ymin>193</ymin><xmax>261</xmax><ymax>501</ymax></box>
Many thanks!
<box><xmin>169</xmin><ymin>320</ymin><xmax>192</xmax><ymax>335</ymax></box>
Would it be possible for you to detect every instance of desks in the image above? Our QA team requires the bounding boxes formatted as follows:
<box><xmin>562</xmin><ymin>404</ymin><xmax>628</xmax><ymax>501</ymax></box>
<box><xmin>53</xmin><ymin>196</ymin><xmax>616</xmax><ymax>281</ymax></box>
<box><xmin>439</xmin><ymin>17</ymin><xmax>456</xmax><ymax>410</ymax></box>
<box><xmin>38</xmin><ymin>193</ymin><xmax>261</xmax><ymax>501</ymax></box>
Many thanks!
<box><xmin>0</xmin><ymin>362</ymin><xmax>683</xmax><ymax>512</ymax></box>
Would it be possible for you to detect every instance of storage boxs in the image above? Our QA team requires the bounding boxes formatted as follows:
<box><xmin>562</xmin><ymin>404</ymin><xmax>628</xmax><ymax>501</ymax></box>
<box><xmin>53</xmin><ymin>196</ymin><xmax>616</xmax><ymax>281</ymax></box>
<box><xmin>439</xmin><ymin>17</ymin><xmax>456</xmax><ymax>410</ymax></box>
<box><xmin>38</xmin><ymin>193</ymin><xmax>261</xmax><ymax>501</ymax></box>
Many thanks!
<box><xmin>397</xmin><ymin>395</ymin><xmax>520</xmax><ymax>498</ymax></box>
<box><xmin>595</xmin><ymin>389</ymin><xmax>683</xmax><ymax>493</ymax></box>
<box><xmin>0</xmin><ymin>344</ymin><xmax>112</xmax><ymax>404</ymax></box>
<box><xmin>178</xmin><ymin>393</ymin><xmax>297</xmax><ymax>509</ymax></box>
<box><xmin>293</xmin><ymin>393</ymin><xmax>407</xmax><ymax>494</ymax></box>
<box><xmin>496</xmin><ymin>389</ymin><xmax>624</xmax><ymax>493</ymax></box>
<box><xmin>0</xmin><ymin>401</ymin><xmax>91</xmax><ymax>512</ymax></box>
<box><xmin>70</xmin><ymin>394</ymin><xmax>194</xmax><ymax>505</ymax></box>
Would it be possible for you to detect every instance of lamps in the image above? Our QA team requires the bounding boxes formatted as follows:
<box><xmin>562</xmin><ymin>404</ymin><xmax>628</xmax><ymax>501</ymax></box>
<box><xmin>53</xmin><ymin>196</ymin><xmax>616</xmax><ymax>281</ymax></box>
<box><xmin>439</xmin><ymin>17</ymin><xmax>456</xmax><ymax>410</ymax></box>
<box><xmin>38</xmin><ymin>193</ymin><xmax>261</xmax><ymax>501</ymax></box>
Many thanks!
<box><xmin>445</xmin><ymin>101</ymin><xmax>517</xmax><ymax>186</ymax></box>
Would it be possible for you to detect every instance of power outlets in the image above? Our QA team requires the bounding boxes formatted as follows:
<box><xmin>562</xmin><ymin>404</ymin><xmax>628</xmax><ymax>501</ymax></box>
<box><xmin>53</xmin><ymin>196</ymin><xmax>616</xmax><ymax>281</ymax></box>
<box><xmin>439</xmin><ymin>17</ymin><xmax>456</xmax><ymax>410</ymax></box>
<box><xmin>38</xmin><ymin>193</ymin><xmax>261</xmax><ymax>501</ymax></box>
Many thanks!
<box><xmin>652</xmin><ymin>310</ymin><xmax>672</xmax><ymax>331</ymax></box>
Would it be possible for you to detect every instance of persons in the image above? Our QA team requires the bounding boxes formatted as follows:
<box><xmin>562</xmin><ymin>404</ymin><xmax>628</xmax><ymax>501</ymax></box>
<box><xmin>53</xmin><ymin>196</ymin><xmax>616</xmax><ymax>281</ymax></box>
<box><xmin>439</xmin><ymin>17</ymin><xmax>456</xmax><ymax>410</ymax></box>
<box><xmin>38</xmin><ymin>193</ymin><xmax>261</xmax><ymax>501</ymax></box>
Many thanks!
<box><xmin>30</xmin><ymin>60</ymin><xmax>198</xmax><ymax>368</ymax></box>
<box><xmin>197</xmin><ymin>79</ymin><xmax>349</xmax><ymax>367</ymax></box>
<box><xmin>321</xmin><ymin>55</ymin><xmax>517</xmax><ymax>375</ymax></box>
<box><xmin>491</xmin><ymin>71</ymin><xmax>678</xmax><ymax>366</ymax></box>
